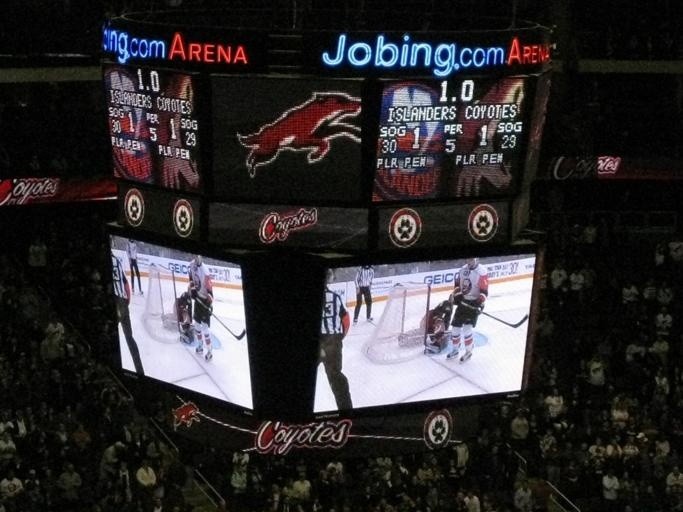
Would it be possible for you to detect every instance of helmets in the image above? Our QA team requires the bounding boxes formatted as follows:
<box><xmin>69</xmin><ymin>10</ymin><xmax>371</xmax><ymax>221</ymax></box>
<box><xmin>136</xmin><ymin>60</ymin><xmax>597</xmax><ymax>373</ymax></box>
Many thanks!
<box><xmin>192</xmin><ymin>255</ymin><xmax>202</xmax><ymax>266</ymax></box>
<box><xmin>466</xmin><ymin>258</ymin><xmax>479</xmax><ymax>270</ymax></box>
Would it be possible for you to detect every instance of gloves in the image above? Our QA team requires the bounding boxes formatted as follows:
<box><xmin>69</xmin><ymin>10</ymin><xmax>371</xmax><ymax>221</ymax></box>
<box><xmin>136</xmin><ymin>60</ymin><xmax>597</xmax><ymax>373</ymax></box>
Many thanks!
<box><xmin>477</xmin><ymin>293</ymin><xmax>486</xmax><ymax>304</ymax></box>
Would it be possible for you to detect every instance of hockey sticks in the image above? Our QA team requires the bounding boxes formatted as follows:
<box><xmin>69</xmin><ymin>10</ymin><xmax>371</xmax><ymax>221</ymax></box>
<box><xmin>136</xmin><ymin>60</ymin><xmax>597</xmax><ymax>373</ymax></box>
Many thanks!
<box><xmin>461</xmin><ymin>301</ymin><xmax>527</xmax><ymax>327</ymax></box>
<box><xmin>195</xmin><ymin>297</ymin><xmax>245</xmax><ymax>340</ymax></box>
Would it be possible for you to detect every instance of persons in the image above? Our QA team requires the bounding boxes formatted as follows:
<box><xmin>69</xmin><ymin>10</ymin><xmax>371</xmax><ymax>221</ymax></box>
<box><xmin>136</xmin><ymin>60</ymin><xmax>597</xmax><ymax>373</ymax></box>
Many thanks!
<box><xmin>1</xmin><ymin>221</ymin><xmax>117</xmax><ymax>369</ymax></box>
<box><xmin>2</xmin><ymin>362</ymin><xmax>230</xmax><ymax>512</ymax></box>
<box><xmin>526</xmin><ymin>220</ymin><xmax>683</xmax><ymax>512</ymax></box>
<box><xmin>231</xmin><ymin>391</ymin><xmax>534</xmax><ymax>512</ymax></box>
<box><xmin>319</xmin><ymin>258</ymin><xmax>488</xmax><ymax>409</ymax></box>
<box><xmin>112</xmin><ymin>238</ymin><xmax>213</xmax><ymax>374</ymax></box>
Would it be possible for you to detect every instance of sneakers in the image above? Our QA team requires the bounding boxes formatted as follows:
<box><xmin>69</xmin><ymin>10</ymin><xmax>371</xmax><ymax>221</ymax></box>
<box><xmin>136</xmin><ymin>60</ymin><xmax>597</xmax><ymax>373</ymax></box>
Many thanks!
<box><xmin>447</xmin><ymin>351</ymin><xmax>472</xmax><ymax>361</ymax></box>
<box><xmin>196</xmin><ymin>345</ymin><xmax>212</xmax><ymax>359</ymax></box>
